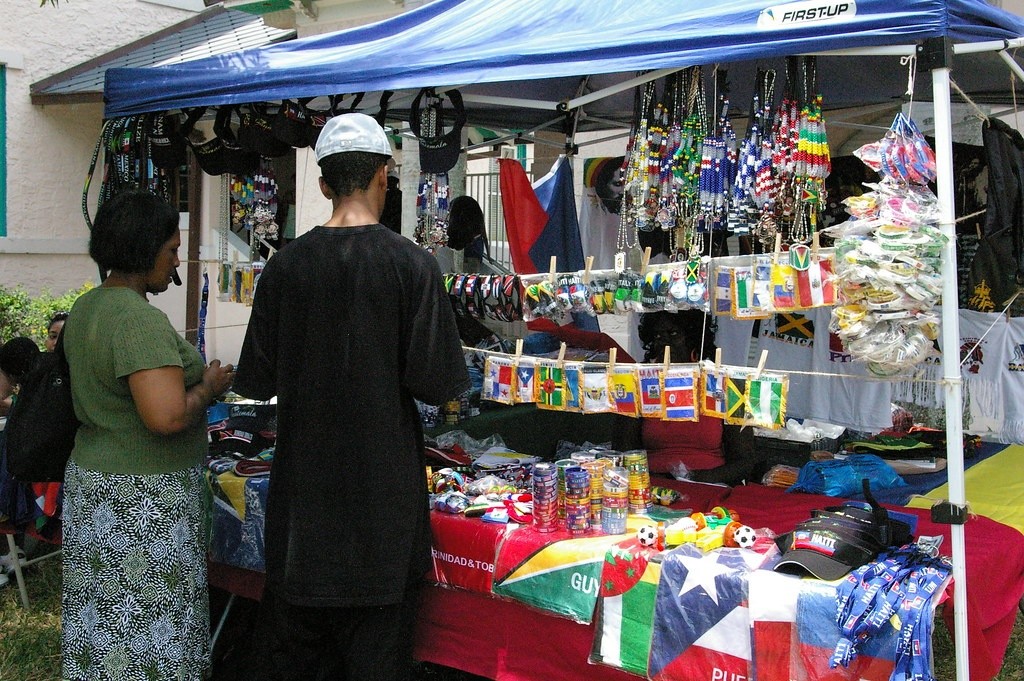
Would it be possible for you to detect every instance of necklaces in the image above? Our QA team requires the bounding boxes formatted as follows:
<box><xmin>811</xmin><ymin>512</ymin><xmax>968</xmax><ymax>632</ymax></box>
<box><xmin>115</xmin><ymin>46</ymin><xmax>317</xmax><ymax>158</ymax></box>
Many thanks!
<box><xmin>613</xmin><ymin>54</ymin><xmax>831</xmax><ymax>277</ymax></box>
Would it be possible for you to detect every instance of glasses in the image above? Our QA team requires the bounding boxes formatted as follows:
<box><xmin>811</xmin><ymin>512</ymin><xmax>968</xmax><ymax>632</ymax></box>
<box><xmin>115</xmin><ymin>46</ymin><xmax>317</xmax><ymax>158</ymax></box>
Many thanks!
<box><xmin>54</xmin><ymin>311</ymin><xmax>70</xmax><ymax>317</ymax></box>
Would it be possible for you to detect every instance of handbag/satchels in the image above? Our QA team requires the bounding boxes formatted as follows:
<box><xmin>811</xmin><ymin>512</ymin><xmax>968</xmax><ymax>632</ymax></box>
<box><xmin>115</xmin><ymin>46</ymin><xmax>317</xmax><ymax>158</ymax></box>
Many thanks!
<box><xmin>5</xmin><ymin>320</ymin><xmax>83</xmax><ymax>483</ymax></box>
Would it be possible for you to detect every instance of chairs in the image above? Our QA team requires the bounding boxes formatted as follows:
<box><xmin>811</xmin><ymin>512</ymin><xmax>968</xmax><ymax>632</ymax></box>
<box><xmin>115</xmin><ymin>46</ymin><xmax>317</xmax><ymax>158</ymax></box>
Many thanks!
<box><xmin>0</xmin><ymin>352</ymin><xmax>80</xmax><ymax>609</ymax></box>
<box><xmin>642</xmin><ymin>416</ymin><xmax>727</xmax><ymax>479</ymax></box>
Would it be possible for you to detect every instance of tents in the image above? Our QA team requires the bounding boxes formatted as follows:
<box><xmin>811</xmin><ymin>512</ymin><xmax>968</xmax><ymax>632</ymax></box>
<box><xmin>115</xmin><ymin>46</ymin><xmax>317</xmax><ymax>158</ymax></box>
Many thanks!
<box><xmin>101</xmin><ymin>0</ymin><xmax>1024</xmax><ymax>681</ymax></box>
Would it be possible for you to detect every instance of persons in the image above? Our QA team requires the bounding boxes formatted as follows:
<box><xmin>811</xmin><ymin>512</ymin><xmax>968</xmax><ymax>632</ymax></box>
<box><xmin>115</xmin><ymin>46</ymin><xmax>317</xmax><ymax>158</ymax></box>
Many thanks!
<box><xmin>60</xmin><ymin>188</ymin><xmax>236</xmax><ymax>681</ymax></box>
<box><xmin>0</xmin><ymin>312</ymin><xmax>79</xmax><ymax>585</ymax></box>
<box><xmin>232</xmin><ymin>112</ymin><xmax>471</xmax><ymax>681</ymax></box>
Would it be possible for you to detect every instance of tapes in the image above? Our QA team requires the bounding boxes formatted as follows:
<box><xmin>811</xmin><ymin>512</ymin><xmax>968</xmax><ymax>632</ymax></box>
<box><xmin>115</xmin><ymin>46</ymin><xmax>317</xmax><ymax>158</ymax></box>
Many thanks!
<box><xmin>532</xmin><ymin>449</ymin><xmax>654</xmax><ymax>535</ymax></box>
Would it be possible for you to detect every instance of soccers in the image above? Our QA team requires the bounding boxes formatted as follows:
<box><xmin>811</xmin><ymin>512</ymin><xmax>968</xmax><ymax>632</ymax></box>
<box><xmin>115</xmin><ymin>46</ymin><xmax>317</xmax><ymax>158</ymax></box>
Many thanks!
<box><xmin>733</xmin><ymin>525</ymin><xmax>757</xmax><ymax>549</ymax></box>
<box><xmin>638</xmin><ymin>525</ymin><xmax>658</xmax><ymax>547</ymax></box>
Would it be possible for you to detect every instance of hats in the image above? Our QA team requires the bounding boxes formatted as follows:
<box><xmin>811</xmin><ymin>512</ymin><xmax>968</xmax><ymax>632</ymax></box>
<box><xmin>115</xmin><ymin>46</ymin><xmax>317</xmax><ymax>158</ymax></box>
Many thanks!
<box><xmin>145</xmin><ymin>91</ymin><xmax>395</xmax><ymax>176</ymax></box>
<box><xmin>447</xmin><ymin>196</ymin><xmax>484</xmax><ymax>250</ymax></box>
<box><xmin>774</xmin><ymin>504</ymin><xmax>915</xmax><ymax>581</ymax></box>
<box><xmin>408</xmin><ymin>87</ymin><xmax>467</xmax><ymax>173</ymax></box>
<box><xmin>315</xmin><ymin>112</ymin><xmax>397</xmax><ymax>170</ymax></box>
<box><xmin>0</xmin><ymin>337</ymin><xmax>52</xmax><ymax>375</ymax></box>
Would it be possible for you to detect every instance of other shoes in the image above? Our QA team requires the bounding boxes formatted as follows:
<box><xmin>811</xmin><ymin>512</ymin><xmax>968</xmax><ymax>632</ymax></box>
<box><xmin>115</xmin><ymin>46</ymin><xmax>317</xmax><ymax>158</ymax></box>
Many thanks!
<box><xmin>0</xmin><ymin>546</ymin><xmax>28</xmax><ymax>590</ymax></box>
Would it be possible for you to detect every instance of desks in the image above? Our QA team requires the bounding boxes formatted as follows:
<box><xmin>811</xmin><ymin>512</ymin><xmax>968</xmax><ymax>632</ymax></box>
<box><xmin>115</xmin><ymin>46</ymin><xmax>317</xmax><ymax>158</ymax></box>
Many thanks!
<box><xmin>201</xmin><ymin>445</ymin><xmax>1024</xmax><ymax>681</ymax></box>
